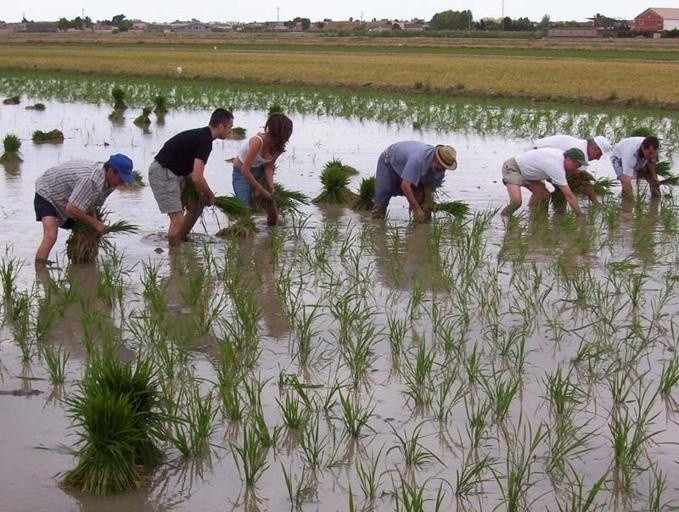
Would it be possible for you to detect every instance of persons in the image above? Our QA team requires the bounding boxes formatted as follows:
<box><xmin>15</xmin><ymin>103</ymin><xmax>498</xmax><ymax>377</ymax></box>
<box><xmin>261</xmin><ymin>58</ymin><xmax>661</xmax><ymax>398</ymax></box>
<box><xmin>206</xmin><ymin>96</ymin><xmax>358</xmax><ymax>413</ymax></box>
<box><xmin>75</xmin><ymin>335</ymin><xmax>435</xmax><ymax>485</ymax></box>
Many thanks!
<box><xmin>232</xmin><ymin>113</ymin><xmax>293</xmax><ymax>227</ymax></box>
<box><xmin>500</xmin><ymin>134</ymin><xmax>611</xmax><ymax>215</ymax></box>
<box><xmin>33</xmin><ymin>154</ymin><xmax>136</xmax><ymax>261</ymax></box>
<box><xmin>370</xmin><ymin>140</ymin><xmax>457</xmax><ymax>222</ymax></box>
<box><xmin>147</xmin><ymin>108</ymin><xmax>235</xmax><ymax>246</ymax></box>
<box><xmin>611</xmin><ymin>136</ymin><xmax>664</xmax><ymax>199</ymax></box>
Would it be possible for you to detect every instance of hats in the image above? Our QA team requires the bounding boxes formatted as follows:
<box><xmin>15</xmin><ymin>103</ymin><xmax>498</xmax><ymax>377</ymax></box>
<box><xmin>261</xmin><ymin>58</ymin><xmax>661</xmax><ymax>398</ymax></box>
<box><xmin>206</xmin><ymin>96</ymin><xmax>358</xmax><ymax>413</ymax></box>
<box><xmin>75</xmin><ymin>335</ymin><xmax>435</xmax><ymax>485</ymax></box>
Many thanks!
<box><xmin>565</xmin><ymin>147</ymin><xmax>589</xmax><ymax>166</ymax></box>
<box><xmin>593</xmin><ymin>135</ymin><xmax>611</xmax><ymax>159</ymax></box>
<box><xmin>110</xmin><ymin>153</ymin><xmax>136</xmax><ymax>184</ymax></box>
<box><xmin>435</xmin><ymin>144</ymin><xmax>458</xmax><ymax>170</ymax></box>
